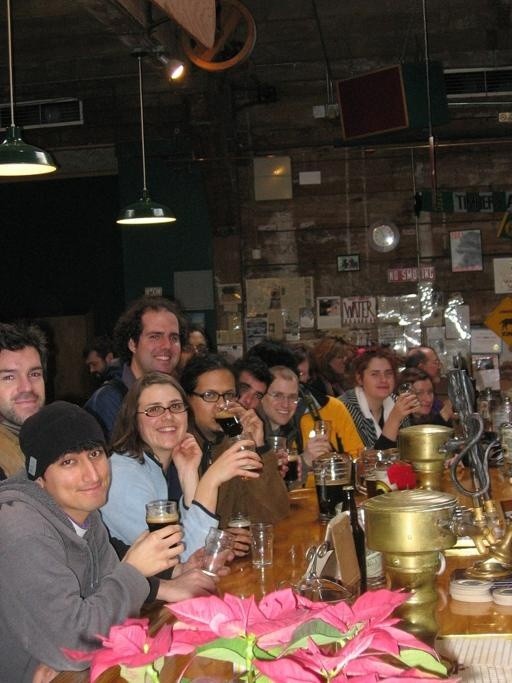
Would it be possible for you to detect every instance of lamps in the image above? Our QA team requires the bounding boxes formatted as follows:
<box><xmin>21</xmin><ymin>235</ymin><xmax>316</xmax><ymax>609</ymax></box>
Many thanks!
<box><xmin>153</xmin><ymin>46</ymin><xmax>184</xmax><ymax>81</ymax></box>
<box><xmin>116</xmin><ymin>199</ymin><xmax>177</xmax><ymax>228</ymax></box>
<box><xmin>1</xmin><ymin>139</ymin><xmax>56</xmax><ymax>179</ymax></box>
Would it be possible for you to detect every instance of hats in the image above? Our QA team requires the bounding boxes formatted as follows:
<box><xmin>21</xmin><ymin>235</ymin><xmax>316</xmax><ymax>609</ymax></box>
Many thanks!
<box><xmin>19</xmin><ymin>400</ymin><xmax>110</xmax><ymax>481</ymax></box>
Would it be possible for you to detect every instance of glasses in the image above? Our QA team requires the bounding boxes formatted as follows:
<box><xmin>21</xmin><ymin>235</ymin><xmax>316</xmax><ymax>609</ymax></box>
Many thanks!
<box><xmin>266</xmin><ymin>391</ymin><xmax>298</xmax><ymax>402</ymax></box>
<box><xmin>135</xmin><ymin>402</ymin><xmax>190</xmax><ymax>417</ymax></box>
<box><xmin>183</xmin><ymin>343</ymin><xmax>208</xmax><ymax>353</ymax></box>
<box><xmin>192</xmin><ymin>390</ymin><xmax>241</xmax><ymax>403</ymax></box>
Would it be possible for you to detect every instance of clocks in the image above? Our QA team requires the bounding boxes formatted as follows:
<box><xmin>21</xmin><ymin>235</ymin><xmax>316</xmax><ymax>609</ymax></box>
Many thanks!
<box><xmin>368</xmin><ymin>219</ymin><xmax>400</xmax><ymax>253</ymax></box>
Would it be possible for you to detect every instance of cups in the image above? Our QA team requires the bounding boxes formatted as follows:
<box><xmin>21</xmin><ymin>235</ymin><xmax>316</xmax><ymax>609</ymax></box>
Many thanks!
<box><xmin>233</xmin><ymin>439</ymin><xmax>260</xmax><ymax>481</ymax></box>
<box><xmin>275</xmin><ymin>453</ymin><xmax>298</xmax><ymax>492</ymax></box>
<box><xmin>353</xmin><ymin>449</ymin><xmax>391</xmax><ymax>502</ymax></box>
<box><xmin>311</xmin><ymin>451</ymin><xmax>353</xmax><ymax>523</ymax></box>
<box><xmin>311</xmin><ymin>420</ymin><xmax>332</xmax><ymax>439</ymax></box>
<box><xmin>228</xmin><ymin>518</ymin><xmax>252</xmax><ymax>557</ymax></box>
<box><xmin>200</xmin><ymin>526</ymin><xmax>235</xmax><ymax>577</ymax></box>
<box><xmin>145</xmin><ymin>499</ymin><xmax>180</xmax><ymax>552</ymax></box>
<box><xmin>210</xmin><ymin>401</ymin><xmax>243</xmax><ymax>438</ymax></box>
<box><xmin>267</xmin><ymin>434</ymin><xmax>288</xmax><ymax>456</ymax></box>
<box><xmin>397</xmin><ymin>382</ymin><xmax>422</xmax><ymax>414</ymax></box>
<box><xmin>249</xmin><ymin>522</ymin><xmax>274</xmax><ymax>567</ymax></box>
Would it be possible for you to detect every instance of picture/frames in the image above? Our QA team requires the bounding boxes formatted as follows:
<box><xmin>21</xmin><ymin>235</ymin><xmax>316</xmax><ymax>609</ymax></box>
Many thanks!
<box><xmin>336</xmin><ymin>255</ymin><xmax>362</xmax><ymax>271</ymax></box>
<box><xmin>450</xmin><ymin>229</ymin><xmax>483</xmax><ymax>273</ymax></box>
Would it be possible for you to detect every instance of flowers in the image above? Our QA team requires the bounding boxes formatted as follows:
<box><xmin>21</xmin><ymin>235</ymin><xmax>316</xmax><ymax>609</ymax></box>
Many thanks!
<box><xmin>58</xmin><ymin>586</ymin><xmax>461</xmax><ymax>683</ymax></box>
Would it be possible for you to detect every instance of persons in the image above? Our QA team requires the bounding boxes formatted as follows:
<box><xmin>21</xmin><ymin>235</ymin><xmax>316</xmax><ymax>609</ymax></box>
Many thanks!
<box><xmin>0</xmin><ymin>399</ymin><xmax>187</xmax><ymax>682</ymax></box>
<box><xmin>81</xmin><ymin>297</ymin><xmax>190</xmax><ymax>446</ymax></box>
<box><xmin>397</xmin><ymin>344</ymin><xmax>441</xmax><ymax>403</ymax></box>
<box><xmin>395</xmin><ymin>368</ymin><xmax>453</xmax><ymax>427</ymax></box>
<box><xmin>246</xmin><ymin>345</ymin><xmax>294</xmax><ymax>379</ymax></box>
<box><xmin>82</xmin><ymin>334</ymin><xmax>122</xmax><ymax>382</ymax></box>
<box><xmin>169</xmin><ymin>324</ymin><xmax>206</xmax><ymax>372</ymax></box>
<box><xmin>96</xmin><ymin>370</ymin><xmax>262</xmax><ymax>579</ymax></box>
<box><xmin>288</xmin><ymin>348</ymin><xmax>368</xmax><ymax>476</ymax></box>
<box><xmin>257</xmin><ymin>364</ymin><xmax>307</xmax><ymax>490</ymax></box>
<box><xmin>0</xmin><ymin>322</ymin><xmax>45</xmax><ymax>485</ymax></box>
<box><xmin>177</xmin><ymin>350</ymin><xmax>289</xmax><ymax>533</ymax></box>
<box><xmin>232</xmin><ymin>358</ymin><xmax>273</xmax><ymax>445</ymax></box>
<box><xmin>336</xmin><ymin>350</ymin><xmax>420</xmax><ymax>452</ymax></box>
